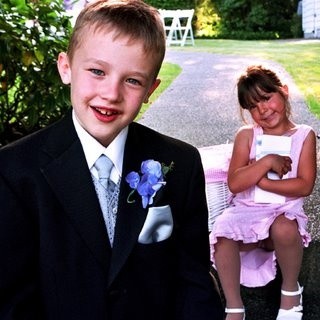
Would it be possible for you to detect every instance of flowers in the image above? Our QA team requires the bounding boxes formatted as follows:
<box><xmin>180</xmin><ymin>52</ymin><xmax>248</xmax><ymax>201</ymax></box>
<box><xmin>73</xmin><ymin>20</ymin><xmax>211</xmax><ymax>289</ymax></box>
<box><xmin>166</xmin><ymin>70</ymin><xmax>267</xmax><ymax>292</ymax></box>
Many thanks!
<box><xmin>125</xmin><ymin>159</ymin><xmax>175</xmax><ymax>208</ymax></box>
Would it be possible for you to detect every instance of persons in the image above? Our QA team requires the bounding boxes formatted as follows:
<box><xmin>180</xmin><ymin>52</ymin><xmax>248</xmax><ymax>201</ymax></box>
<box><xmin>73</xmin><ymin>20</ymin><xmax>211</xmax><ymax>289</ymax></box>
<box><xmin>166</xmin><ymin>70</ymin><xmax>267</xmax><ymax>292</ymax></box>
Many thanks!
<box><xmin>1</xmin><ymin>0</ymin><xmax>226</xmax><ymax>319</ymax></box>
<box><xmin>207</xmin><ymin>64</ymin><xmax>318</xmax><ymax>320</ymax></box>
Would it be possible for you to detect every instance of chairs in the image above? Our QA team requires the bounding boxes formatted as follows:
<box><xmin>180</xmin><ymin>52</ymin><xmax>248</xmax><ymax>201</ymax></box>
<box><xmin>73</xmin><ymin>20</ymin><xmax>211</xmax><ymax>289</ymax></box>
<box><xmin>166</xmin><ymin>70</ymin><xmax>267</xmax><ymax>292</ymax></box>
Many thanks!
<box><xmin>159</xmin><ymin>9</ymin><xmax>195</xmax><ymax>48</ymax></box>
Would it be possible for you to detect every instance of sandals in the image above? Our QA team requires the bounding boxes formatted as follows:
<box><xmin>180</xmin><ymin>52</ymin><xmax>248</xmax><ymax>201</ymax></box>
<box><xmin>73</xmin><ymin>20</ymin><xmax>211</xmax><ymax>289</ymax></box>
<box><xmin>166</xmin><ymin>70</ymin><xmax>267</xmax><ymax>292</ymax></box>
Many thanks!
<box><xmin>276</xmin><ymin>281</ymin><xmax>304</xmax><ymax>320</ymax></box>
<box><xmin>225</xmin><ymin>307</ymin><xmax>246</xmax><ymax>320</ymax></box>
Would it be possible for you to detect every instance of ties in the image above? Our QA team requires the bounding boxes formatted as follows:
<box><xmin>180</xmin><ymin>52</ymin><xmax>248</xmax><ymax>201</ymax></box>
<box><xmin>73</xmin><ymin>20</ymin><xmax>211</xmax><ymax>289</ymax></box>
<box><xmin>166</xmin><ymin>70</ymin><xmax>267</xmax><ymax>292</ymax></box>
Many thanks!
<box><xmin>94</xmin><ymin>155</ymin><xmax>114</xmax><ymax>189</ymax></box>
